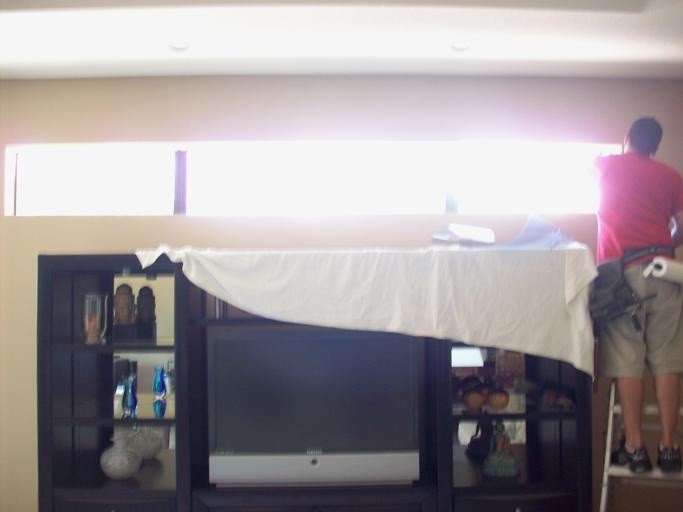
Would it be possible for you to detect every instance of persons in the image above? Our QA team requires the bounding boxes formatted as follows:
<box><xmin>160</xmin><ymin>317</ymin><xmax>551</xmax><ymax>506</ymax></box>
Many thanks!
<box><xmin>593</xmin><ymin>117</ymin><xmax>683</xmax><ymax>474</ymax></box>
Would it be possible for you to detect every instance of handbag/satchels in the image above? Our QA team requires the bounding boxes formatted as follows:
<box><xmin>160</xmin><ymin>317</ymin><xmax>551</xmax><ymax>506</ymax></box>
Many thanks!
<box><xmin>588</xmin><ymin>259</ymin><xmax>638</xmax><ymax>320</ymax></box>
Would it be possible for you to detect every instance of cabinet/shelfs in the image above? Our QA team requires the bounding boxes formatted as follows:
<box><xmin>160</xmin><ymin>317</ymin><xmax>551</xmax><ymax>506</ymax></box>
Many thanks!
<box><xmin>37</xmin><ymin>253</ymin><xmax>592</xmax><ymax>512</ymax></box>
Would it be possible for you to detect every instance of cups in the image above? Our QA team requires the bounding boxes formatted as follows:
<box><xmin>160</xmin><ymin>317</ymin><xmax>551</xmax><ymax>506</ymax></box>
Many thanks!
<box><xmin>83</xmin><ymin>292</ymin><xmax>109</xmax><ymax>346</ymax></box>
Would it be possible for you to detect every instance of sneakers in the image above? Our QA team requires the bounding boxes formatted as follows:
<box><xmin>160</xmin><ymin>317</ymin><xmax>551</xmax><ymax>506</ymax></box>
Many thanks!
<box><xmin>657</xmin><ymin>444</ymin><xmax>683</xmax><ymax>472</ymax></box>
<box><xmin>610</xmin><ymin>435</ymin><xmax>653</xmax><ymax>473</ymax></box>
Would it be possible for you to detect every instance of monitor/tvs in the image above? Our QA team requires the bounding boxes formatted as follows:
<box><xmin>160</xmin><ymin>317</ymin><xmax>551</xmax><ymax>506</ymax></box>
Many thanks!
<box><xmin>206</xmin><ymin>320</ymin><xmax>422</xmax><ymax>492</ymax></box>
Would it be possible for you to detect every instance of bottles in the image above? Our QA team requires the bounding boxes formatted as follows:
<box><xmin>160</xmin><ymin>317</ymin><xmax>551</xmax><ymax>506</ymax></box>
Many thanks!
<box><xmin>121</xmin><ymin>360</ymin><xmax>138</xmax><ymax>393</ymax></box>
<box><xmin>120</xmin><ymin>394</ymin><xmax>138</xmax><ymax>421</ymax></box>
<box><xmin>153</xmin><ymin>363</ymin><xmax>166</xmax><ymax>400</ymax></box>
<box><xmin>152</xmin><ymin>397</ymin><xmax>167</xmax><ymax>418</ymax></box>
